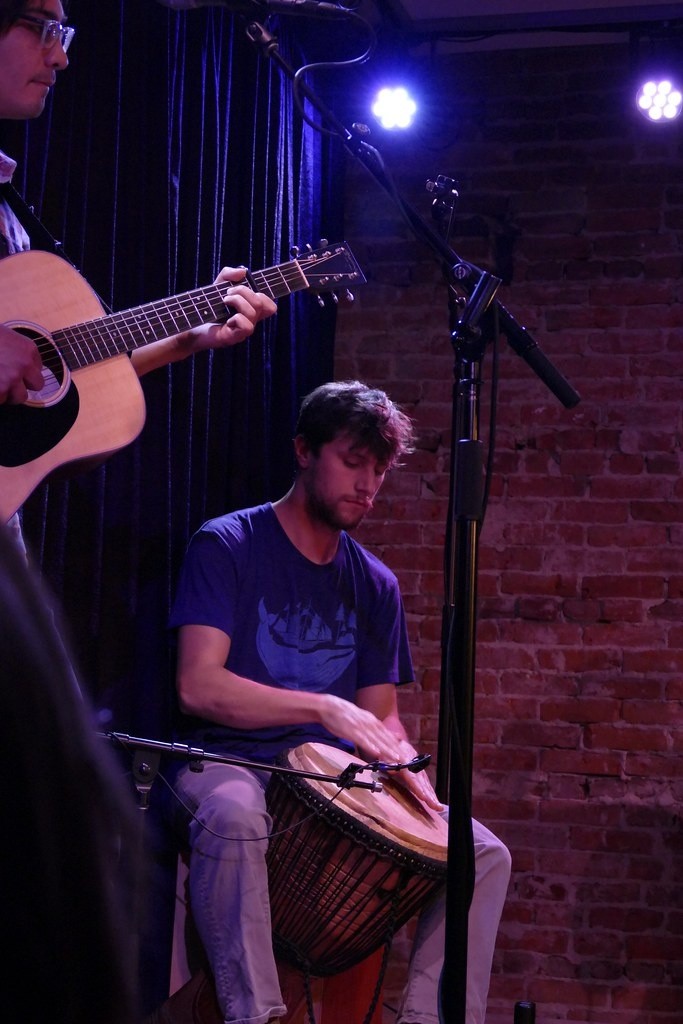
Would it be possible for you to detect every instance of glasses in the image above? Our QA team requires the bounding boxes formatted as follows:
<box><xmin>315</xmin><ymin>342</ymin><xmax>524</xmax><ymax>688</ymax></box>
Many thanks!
<box><xmin>8</xmin><ymin>12</ymin><xmax>76</xmax><ymax>54</ymax></box>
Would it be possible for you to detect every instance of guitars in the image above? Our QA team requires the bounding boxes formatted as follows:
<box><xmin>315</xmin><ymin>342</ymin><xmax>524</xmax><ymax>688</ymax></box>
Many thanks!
<box><xmin>1</xmin><ymin>235</ymin><xmax>372</xmax><ymax>532</ymax></box>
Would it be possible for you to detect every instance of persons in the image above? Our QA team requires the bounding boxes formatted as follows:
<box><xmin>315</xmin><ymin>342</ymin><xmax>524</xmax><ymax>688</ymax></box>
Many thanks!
<box><xmin>0</xmin><ymin>0</ymin><xmax>278</xmax><ymax>1024</ymax></box>
<box><xmin>162</xmin><ymin>380</ymin><xmax>512</xmax><ymax>1024</ymax></box>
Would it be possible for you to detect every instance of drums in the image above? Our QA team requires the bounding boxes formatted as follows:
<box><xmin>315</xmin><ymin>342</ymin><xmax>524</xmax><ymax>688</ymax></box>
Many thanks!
<box><xmin>144</xmin><ymin>740</ymin><xmax>452</xmax><ymax>1024</ymax></box>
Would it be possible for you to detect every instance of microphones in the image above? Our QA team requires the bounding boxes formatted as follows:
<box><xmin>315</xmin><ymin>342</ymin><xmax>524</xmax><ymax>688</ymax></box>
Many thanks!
<box><xmin>408</xmin><ymin>754</ymin><xmax>432</xmax><ymax>773</ymax></box>
<box><xmin>158</xmin><ymin>0</ymin><xmax>355</xmax><ymax>20</ymax></box>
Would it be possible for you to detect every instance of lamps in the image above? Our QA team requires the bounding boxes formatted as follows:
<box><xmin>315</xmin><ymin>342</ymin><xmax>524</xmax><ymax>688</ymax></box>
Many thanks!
<box><xmin>627</xmin><ymin>19</ymin><xmax>683</xmax><ymax>127</ymax></box>
